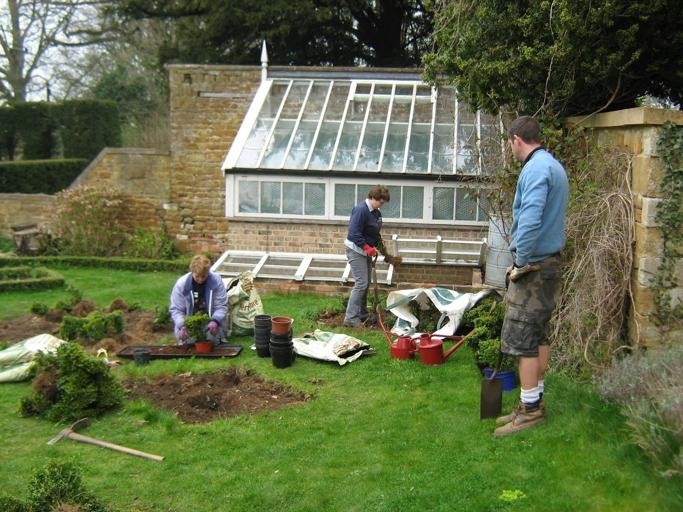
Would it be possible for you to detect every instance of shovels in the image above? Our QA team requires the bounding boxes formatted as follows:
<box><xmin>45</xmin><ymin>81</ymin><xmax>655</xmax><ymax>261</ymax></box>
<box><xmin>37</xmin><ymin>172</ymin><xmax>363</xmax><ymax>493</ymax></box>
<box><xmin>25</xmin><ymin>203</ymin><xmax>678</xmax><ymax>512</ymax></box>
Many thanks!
<box><xmin>481</xmin><ymin>342</ymin><xmax>504</xmax><ymax>418</ymax></box>
<box><xmin>366</xmin><ymin>249</ymin><xmax>384</xmax><ymax>331</ymax></box>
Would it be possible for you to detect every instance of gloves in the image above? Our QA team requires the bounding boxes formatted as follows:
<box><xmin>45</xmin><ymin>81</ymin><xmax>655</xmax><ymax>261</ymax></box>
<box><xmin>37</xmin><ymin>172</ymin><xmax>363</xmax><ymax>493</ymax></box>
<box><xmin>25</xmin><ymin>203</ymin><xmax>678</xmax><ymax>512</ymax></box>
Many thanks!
<box><xmin>179</xmin><ymin>326</ymin><xmax>188</xmax><ymax>339</ymax></box>
<box><xmin>208</xmin><ymin>321</ymin><xmax>218</xmax><ymax>334</ymax></box>
<box><xmin>510</xmin><ymin>265</ymin><xmax>540</xmax><ymax>282</ymax></box>
<box><xmin>363</xmin><ymin>244</ymin><xmax>376</xmax><ymax>256</ymax></box>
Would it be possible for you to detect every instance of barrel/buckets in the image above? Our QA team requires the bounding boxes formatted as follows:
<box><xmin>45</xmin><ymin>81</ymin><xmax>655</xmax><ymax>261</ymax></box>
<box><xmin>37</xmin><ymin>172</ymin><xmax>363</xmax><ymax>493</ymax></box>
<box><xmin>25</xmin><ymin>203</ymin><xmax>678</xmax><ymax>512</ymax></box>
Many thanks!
<box><xmin>485</xmin><ymin>212</ymin><xmax>515</xmax><ymax>289</ymax></box>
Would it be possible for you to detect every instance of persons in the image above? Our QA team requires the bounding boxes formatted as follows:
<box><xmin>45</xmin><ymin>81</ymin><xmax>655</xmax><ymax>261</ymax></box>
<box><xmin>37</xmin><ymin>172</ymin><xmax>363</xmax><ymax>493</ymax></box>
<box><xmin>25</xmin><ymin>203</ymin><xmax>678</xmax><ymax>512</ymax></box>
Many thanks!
<box><xmin>493</xmin><ymin>115</ymin><xmax>570</xmax><ymax>437</ymax></box>
<box><xmin>170</xmin><ymin>253</ymin><xmax>230</xmax><ymax>344</ymax></box>
<box><xmin>343</xmin><ymin>184</ymin><xmax>391</xmax><ymax>328</ymax></box>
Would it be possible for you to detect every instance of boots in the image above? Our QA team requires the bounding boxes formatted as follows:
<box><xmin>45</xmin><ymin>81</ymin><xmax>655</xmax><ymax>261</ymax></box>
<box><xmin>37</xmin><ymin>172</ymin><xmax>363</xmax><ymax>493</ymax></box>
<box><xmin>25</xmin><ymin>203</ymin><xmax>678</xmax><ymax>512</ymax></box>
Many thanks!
<box><xmin>495</xmin><ymin>392</ymin><xmax>545</xmax><ymax>424</ymax></box>
<box><xmin>495</xmin><ymin>402</ymin><xmax>544</xmax><ymax>435</ymax></box>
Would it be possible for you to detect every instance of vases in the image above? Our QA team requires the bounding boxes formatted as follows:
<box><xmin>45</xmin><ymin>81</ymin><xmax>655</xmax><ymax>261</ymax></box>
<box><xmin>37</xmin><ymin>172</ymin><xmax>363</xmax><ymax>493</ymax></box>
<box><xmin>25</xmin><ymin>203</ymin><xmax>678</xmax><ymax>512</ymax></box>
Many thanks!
<box><xmin>254</xmin><ymin>315</ymin><xmax>296</xmax><ymax>369</ymax></box>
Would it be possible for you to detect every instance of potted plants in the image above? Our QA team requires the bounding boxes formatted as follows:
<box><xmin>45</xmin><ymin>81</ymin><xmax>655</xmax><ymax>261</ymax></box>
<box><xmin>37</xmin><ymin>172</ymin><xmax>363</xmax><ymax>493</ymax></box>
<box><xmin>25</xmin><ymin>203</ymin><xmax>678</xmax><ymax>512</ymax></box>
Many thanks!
<box><xmin>467</xmin><ymin>327</ymin><xmax>492</xmax><ymax>376</ymax></box>
<box><xmin>478</xmin><ymin>339</ymin><xmax>517</xmax><ymax>391</ymax></box>
<box><xmin>184</xmin><ymin>313</ymin><xmax>213</xmax><ymax>354</ymax></box>
